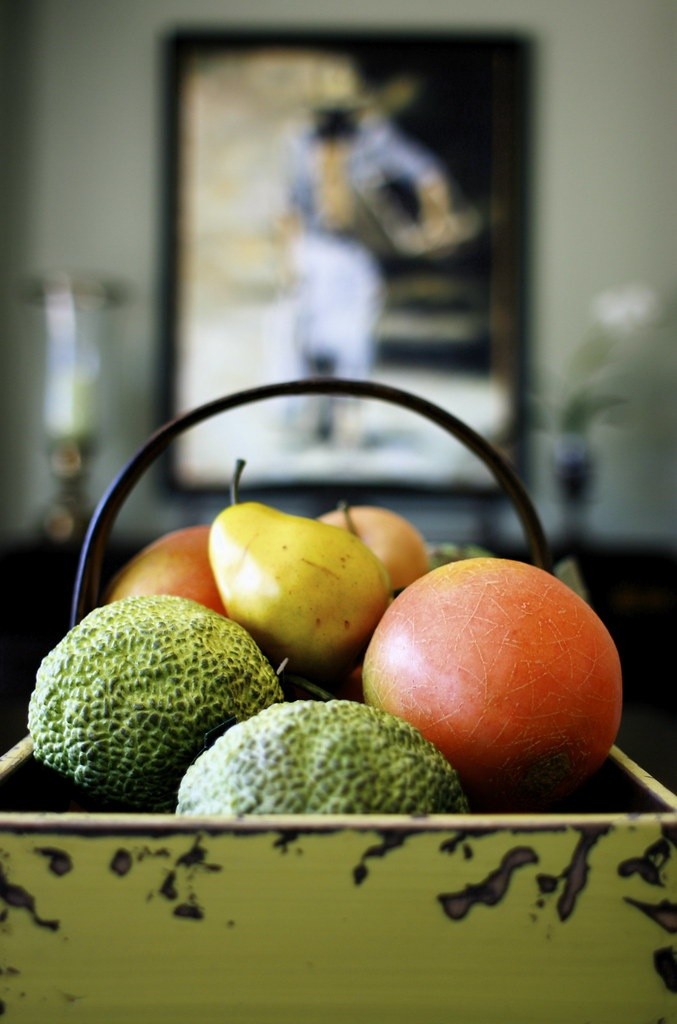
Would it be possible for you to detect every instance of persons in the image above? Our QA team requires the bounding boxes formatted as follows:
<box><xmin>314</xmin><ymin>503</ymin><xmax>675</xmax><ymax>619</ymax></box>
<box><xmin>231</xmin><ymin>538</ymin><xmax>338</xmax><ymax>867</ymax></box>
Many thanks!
<box><xmin>278</xmin><ymin>98</ymin><xmax>452</xmax><ymax>457</ymax></box>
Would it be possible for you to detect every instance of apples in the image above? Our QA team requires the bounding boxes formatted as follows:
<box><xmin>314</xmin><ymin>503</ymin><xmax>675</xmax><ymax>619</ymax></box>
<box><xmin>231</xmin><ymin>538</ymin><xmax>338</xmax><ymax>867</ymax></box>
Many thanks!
<box><xmin>311</xmin><ymin>503</ymin><xmax>428</xmax><ymax>591</ymax></box>
<box><xmin>97</xmin><ymin>525</ymin><xmax>226</xmax><ymax>614</ymax></box>
<box><xmin>361</xmin><ymin>556</ymin><xmax>622</xmax><ymax>811</ymax></box>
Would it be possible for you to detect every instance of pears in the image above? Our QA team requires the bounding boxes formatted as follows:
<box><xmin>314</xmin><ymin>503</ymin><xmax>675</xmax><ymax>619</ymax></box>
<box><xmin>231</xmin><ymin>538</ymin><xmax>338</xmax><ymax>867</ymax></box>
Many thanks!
<box><xmin>208</xmin><ymin>457</ymin><xmax>389</xmax><ymax>685</ymax></box>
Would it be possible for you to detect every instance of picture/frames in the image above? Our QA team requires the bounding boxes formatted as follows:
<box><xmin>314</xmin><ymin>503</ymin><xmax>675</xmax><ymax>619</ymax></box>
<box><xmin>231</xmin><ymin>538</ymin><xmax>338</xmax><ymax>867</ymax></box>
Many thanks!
<box><xmin>155</xmin><ymin>23</ymin><xmax>535</xmax><ymax>500</ymax></box>
